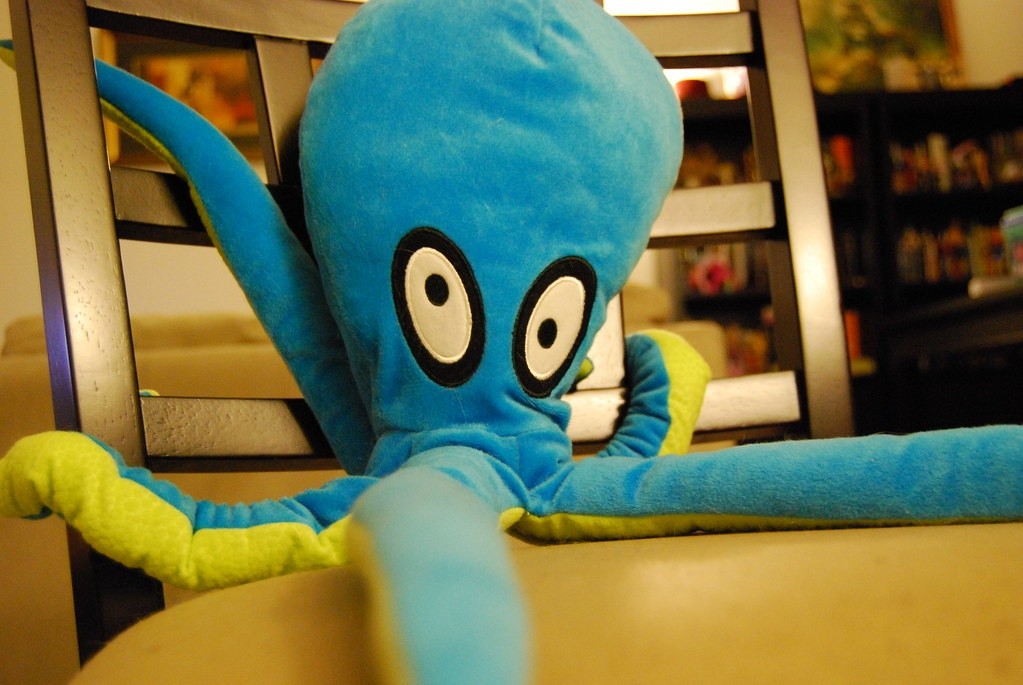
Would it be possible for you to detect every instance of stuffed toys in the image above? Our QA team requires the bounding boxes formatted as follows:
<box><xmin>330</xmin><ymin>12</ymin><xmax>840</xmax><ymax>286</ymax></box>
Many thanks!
<box><xmin>1</xmin><ymin>1</ymin><xmax>1023</xmax><ymax>683</ymax></box>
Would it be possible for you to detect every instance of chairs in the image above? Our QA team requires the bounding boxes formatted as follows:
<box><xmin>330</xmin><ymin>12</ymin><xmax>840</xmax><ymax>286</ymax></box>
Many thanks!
<box><xmin>8</xmin><ymin>2</ymin><xmax>1022</xmax><ymax>684</ymax></box>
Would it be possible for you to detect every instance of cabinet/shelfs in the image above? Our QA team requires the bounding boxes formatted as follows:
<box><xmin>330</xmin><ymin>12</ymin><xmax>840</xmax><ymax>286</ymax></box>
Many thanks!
<box><xmin>680</xmin><ymin>75</ymin><xmax>1022</xmax><ymax>369</ymax></box>
